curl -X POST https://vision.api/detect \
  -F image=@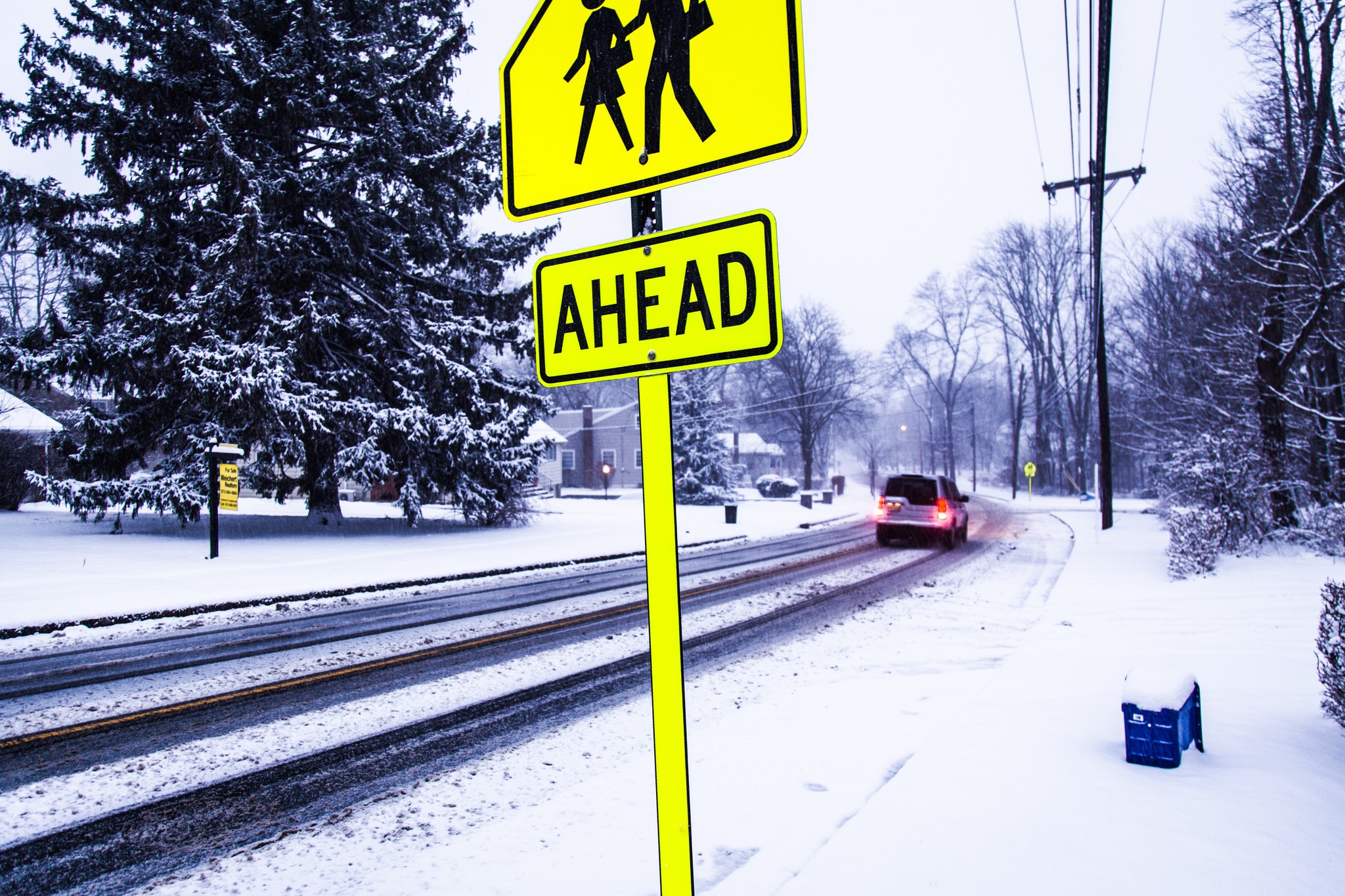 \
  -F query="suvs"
[875,473,969,551]
